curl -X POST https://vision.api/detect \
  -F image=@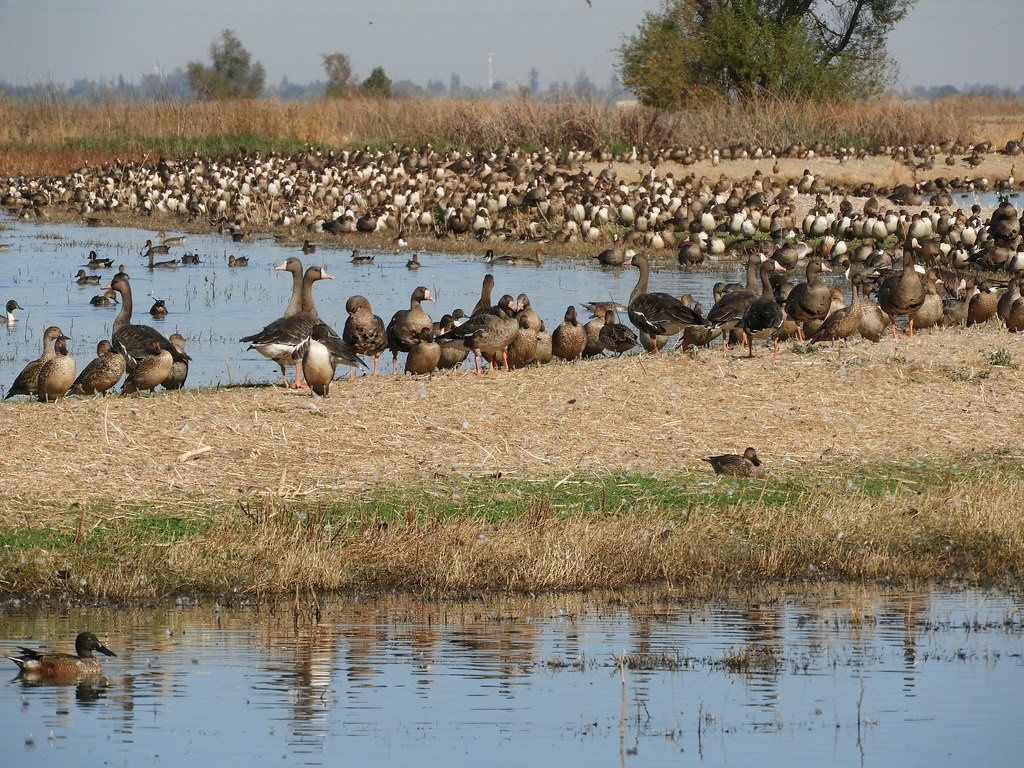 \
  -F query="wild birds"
[701,447,762,478]
[7,632,118,685]
[0,139,1024,406]
